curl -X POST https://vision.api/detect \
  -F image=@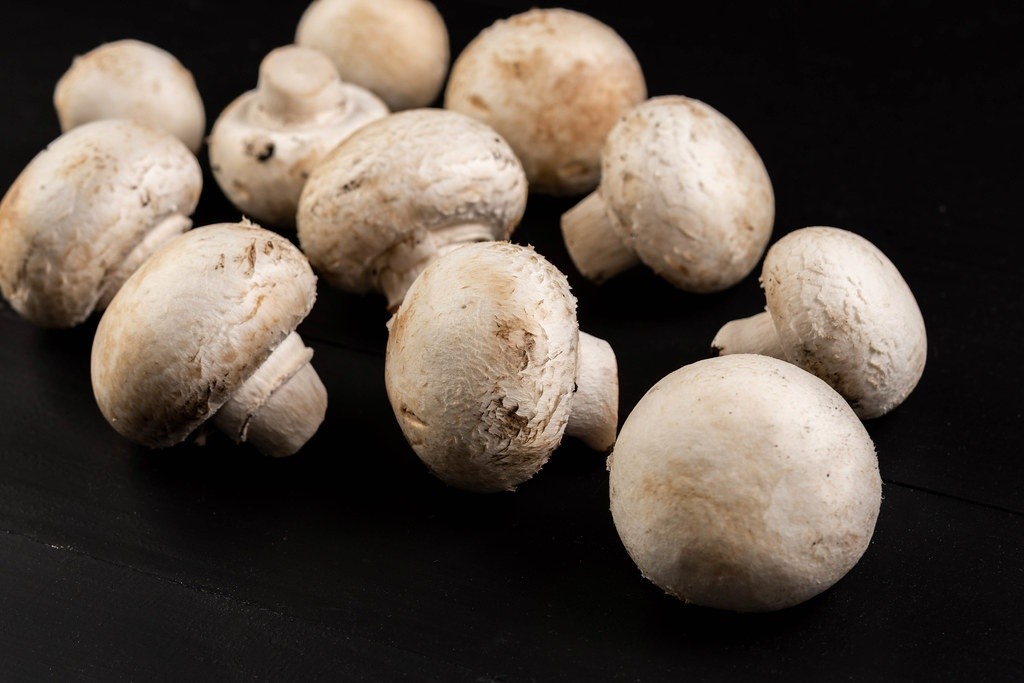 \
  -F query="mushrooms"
[1,0,928,611]
